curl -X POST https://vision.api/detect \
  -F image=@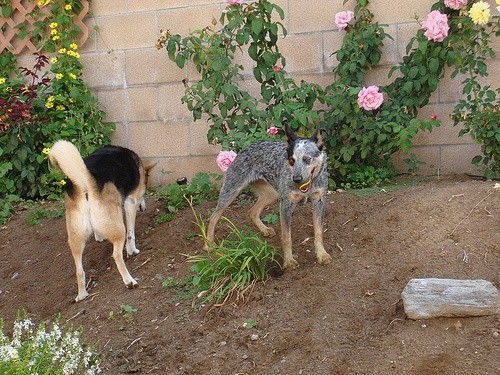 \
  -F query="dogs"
[48,139,157,303]
[202,121,334,271]
